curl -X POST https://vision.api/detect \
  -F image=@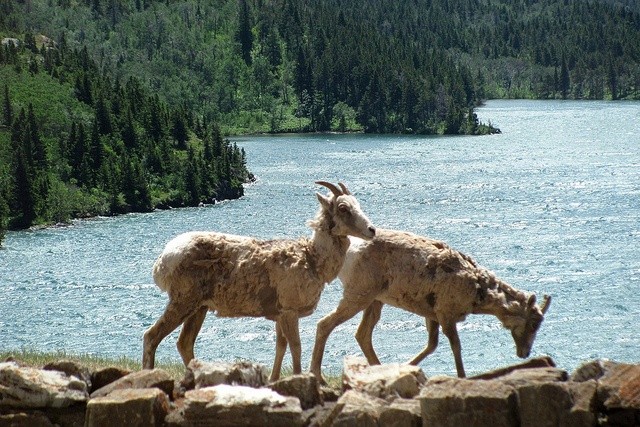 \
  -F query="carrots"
[141,179,377,384]
[309,229,553,386]
